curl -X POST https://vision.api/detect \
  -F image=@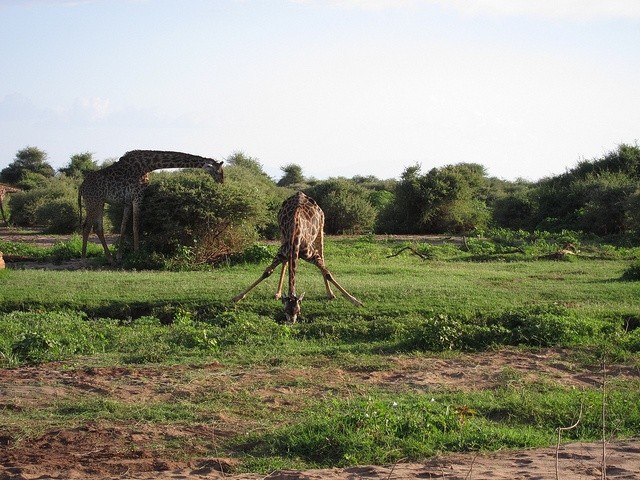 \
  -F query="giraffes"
[77,149,224,266]
[231,190,366,325]
